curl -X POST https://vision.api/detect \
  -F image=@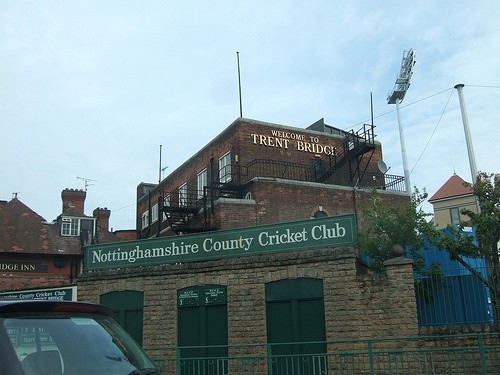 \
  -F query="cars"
[1,298,165,375]
